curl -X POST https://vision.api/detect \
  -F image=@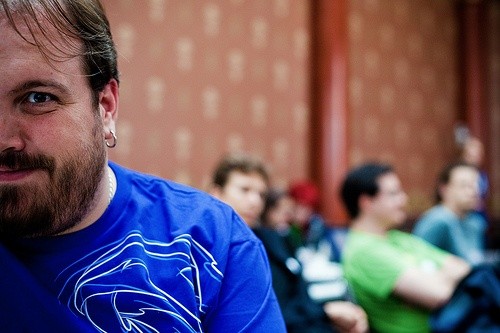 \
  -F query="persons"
[210,129,500,333]
[0,0,289,333]
